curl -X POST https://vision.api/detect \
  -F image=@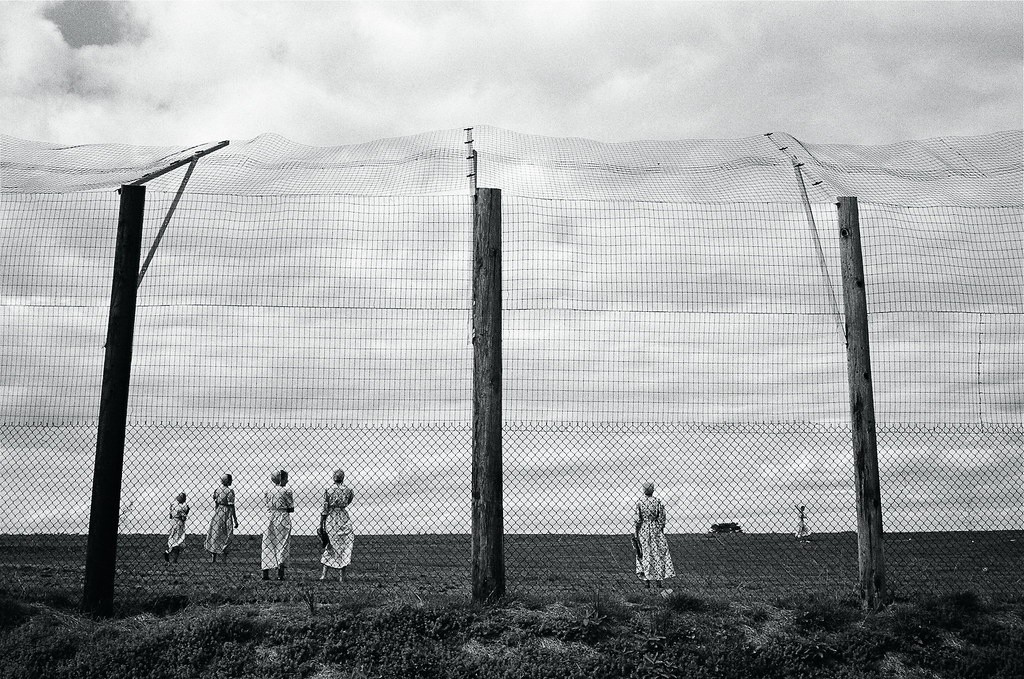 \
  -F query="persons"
[630,482,675,589]
[261,470,295,581]
[317,469,354,583]
[795,506,810,544]
[165,492,190,563]
[205,474,238,563]
